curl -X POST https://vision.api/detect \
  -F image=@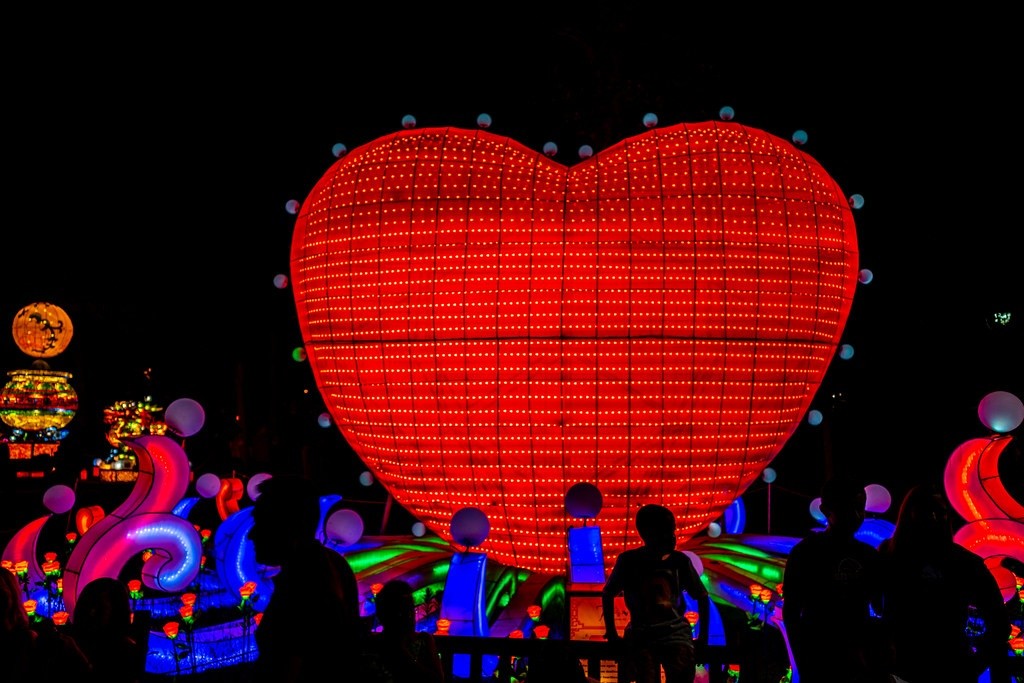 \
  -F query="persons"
[0,565,142,683]
[246,472,361,683]
[602,503,711,683]
[783,475,1011,683]
[361,579,446,683]
